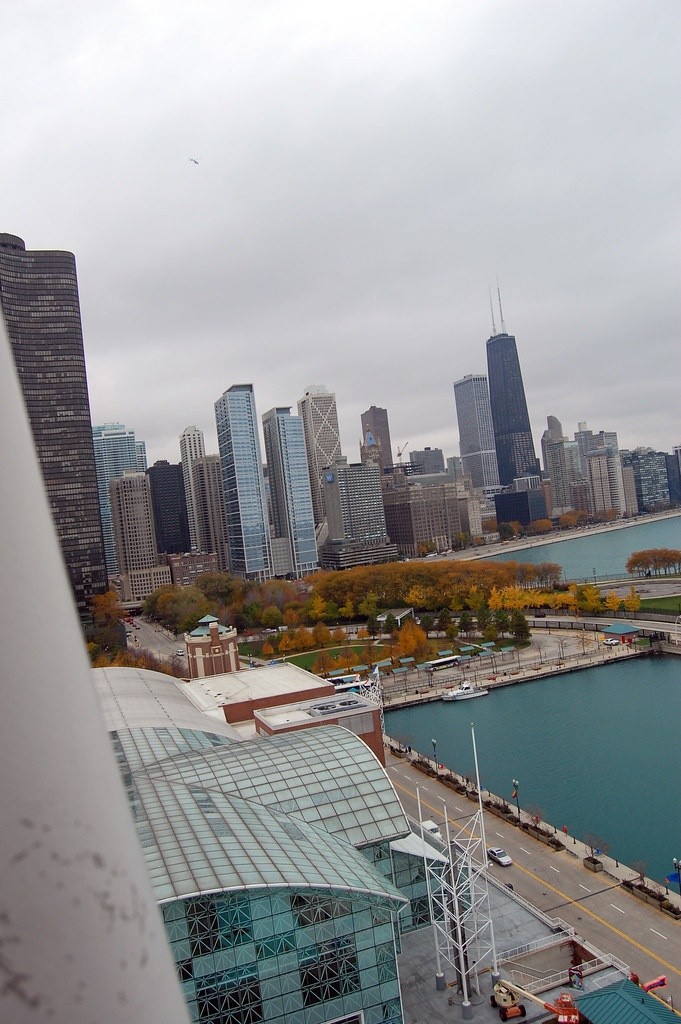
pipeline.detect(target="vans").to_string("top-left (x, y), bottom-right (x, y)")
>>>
top-left (176, 649), bottom-right (185, 656)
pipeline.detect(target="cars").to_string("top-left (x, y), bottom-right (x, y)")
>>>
top-left (487, 846), bottom-right (512, 867)
top-left (603, 639), bottom-right (620, 646)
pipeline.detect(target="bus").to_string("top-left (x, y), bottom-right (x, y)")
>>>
top-left (425, 655), bottom-right (462, 672)
top-left (326, 673), bottom-right (361, 687)
top-left (334, 678), bottom-right (376, 694)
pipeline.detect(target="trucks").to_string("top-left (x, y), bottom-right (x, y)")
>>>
top-left (421, 819), bottom-right (443, 842)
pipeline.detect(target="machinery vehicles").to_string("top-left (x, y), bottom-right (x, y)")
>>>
top-left (489, 978), bottom-right (580, 1024)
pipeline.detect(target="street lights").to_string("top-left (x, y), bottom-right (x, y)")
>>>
top-left (512, 778), bottom-right (520, 813)
top-left (672, 857), bottom-right (681, 897)
top-left (432, 739), bottom-right (437, 762)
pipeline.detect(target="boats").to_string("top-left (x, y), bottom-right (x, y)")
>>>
top-left (440, 680), bottom-right (489, 702)
